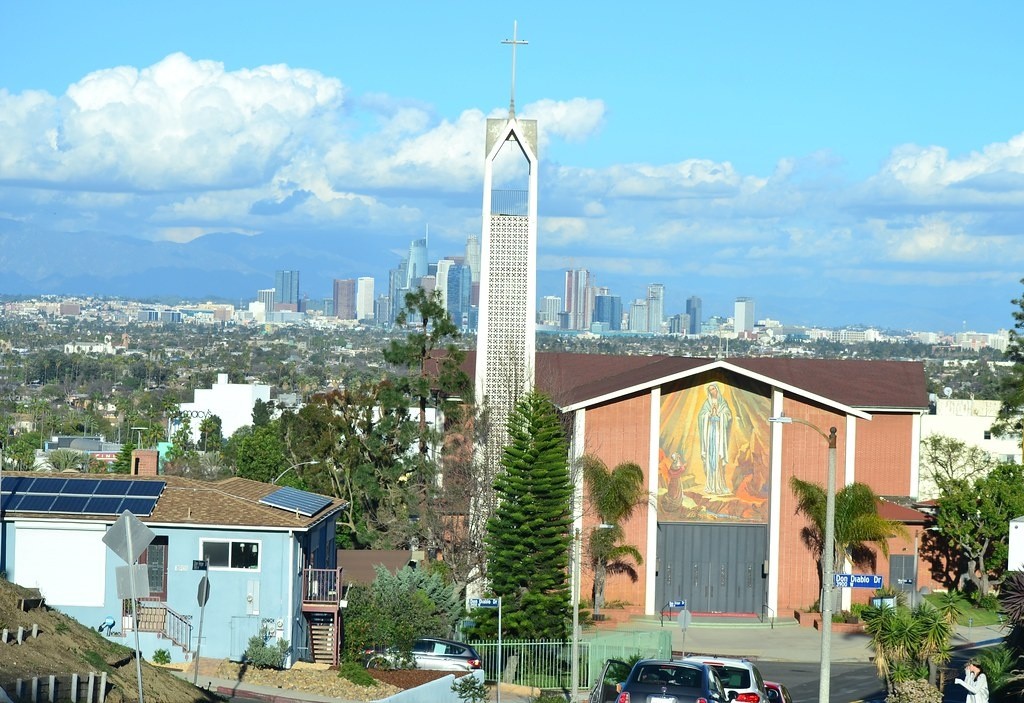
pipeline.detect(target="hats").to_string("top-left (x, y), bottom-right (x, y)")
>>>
top-left (963, 658), bottom-right (981, 666)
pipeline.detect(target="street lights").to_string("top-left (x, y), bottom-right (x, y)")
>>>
top-left (908, 523), bottom-right (944, 608)
top-left (768, 411), bottom-right (840, 703)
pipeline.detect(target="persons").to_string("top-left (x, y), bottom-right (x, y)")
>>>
top-left (616, 669), bottom-right (654, 693)
top-left (954, 657), bottom-right (989, 703)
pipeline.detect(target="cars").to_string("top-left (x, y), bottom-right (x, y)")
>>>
top-left (587, 657), bottom-right (739, 703)
top-left (762, 679), bottom-right (794, 703)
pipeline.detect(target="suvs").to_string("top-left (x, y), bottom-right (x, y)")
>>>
top-left (684, 655), bottom-right (771, 703)
top-left (361, 638), bottom-right (484, 688)
